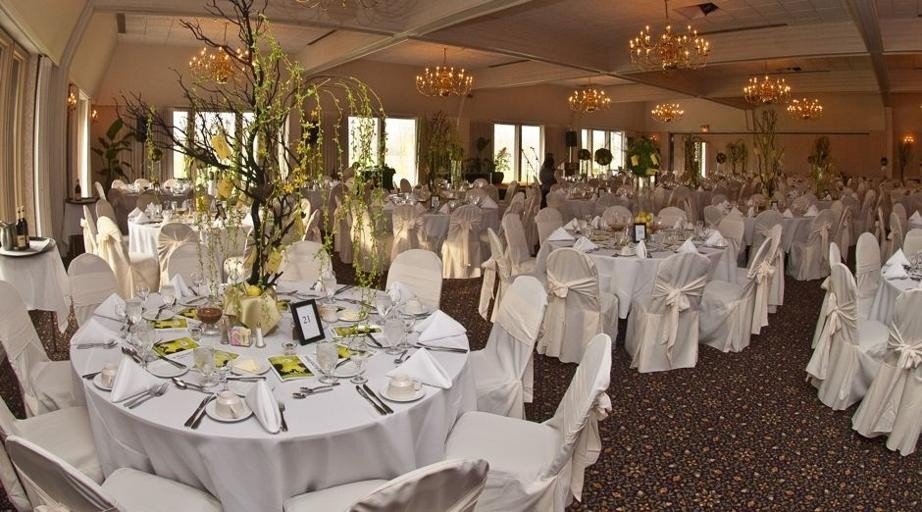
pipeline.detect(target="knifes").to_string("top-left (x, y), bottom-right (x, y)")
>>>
top-left (183, 392), bottom-right (219, 429)
top-left (413, 342), bottom-right (468, 353)
top-left (355, 383), bottom-right (394, 417)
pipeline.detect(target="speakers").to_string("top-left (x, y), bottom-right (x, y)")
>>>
top-left (566, 131), bottom-right (576, 147)
top-left (301, 121), bottom-right (319, 143)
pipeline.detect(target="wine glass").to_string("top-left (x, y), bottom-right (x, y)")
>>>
top-left (115, 268), bottom-right (416, 396)
top-left (579, 214), bottom-right (712, 250)
top-left (145, 197), bottom-right (239, 232)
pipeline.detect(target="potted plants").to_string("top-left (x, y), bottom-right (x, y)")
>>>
top-left (117, 0), bottom-right (394, 337)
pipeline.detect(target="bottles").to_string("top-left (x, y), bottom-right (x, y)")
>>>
top-left (16, 205), bottom-right (30, 250)
top-left (75, 179), bottom-right (81, 201)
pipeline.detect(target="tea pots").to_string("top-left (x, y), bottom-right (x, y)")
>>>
top-left (0, 221), bottom-right (18, 252)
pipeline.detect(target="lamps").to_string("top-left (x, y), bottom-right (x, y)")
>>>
top-left (188, 23), bottom-right (257, 82)
top-left (66, 89), bottom-right (79, 113)
top-left (743, 58), bottom-right (823, 122)
top-left (628, 2), bottom-right (713, 72)
top-left (415, 47), bottom-right (475, 97)
top-left (651, 100), bottom-right (687, 125)
top-left (567, 81), bottom-right (612, 114)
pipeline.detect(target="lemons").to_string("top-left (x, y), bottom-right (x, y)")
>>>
top-left (633, 209), bottom-right (654, 235)
top-left (245, 285), bottom-right (263, 298)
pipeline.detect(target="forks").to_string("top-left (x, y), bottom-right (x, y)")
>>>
top-left (74, 341), bottom-right (118, 350)
top-left (122, 381), bottom-right (169, 410)
top-left (276, 404), bottom-right (290, 431)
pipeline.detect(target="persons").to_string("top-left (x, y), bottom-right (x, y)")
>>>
top-left (539, 156), bottom-right (557, 209)
top-left (340, 161), bottom-right (360, 183)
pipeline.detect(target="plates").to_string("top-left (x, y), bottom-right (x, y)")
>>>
top-left (140, 286), bottom-right (430, 422)
